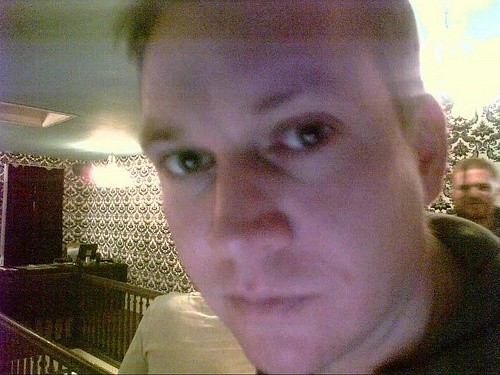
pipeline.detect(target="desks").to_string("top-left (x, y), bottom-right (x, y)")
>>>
top-left (1, 263), bottom-right (128, 318)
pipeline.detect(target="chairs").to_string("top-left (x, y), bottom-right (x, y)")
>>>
top-left (27, 248), bottom-right (55, 265)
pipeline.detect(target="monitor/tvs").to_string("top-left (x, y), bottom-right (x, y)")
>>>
top-left (76, 243), bottom-right (98, 263)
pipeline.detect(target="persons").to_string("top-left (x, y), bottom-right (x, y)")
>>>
top-left (114, 0), bottom-right (500, 375)
top-left (446, 157), bottom-right (500, 237)
top-left (118, 291), bottom-right (258, 375)
